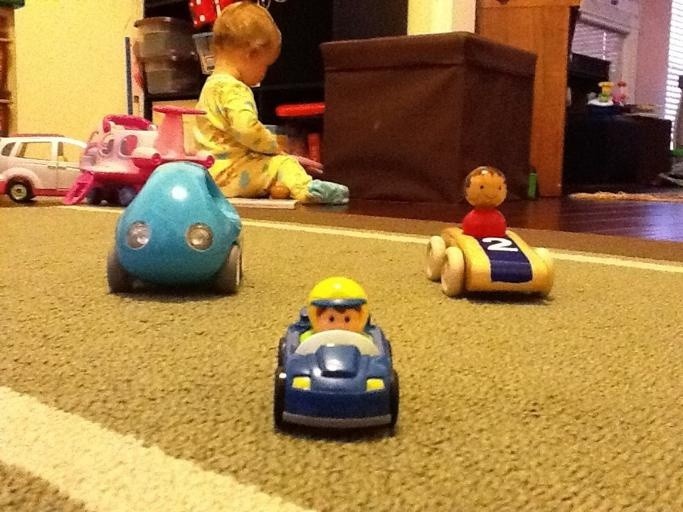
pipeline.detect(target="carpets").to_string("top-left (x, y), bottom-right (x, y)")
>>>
top-left (570, 189), bottom-right (682, 203)
top-left (3, 205), bottom-right (681, 512)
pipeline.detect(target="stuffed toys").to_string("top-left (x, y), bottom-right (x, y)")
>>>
top-left (598, 80), bottom-right (630, 107)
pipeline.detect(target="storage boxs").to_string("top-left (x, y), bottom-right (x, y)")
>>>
top-left (193, 32), bottom-right (221, 70)
top-left (143, 60), bottom-right (192, 93)
top-left (136, 17), bottom-right (189, 58)
top-left (322, 31), bottom-right (538, 205)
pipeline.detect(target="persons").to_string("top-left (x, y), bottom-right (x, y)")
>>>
top-left (191, 2), bottom-right (349, 205)
top-left (424, 166), bottom-right (554, 298)
top-left (275, 276), bottom-right (400, 436)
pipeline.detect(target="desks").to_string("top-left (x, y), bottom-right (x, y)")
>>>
top-left (567, 113), bottom-right (671, 192)
